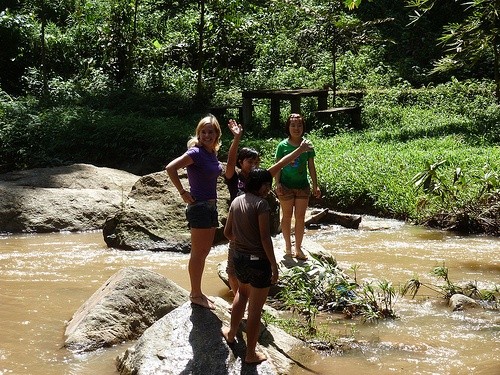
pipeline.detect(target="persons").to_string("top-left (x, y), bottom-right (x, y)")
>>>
top-left (224, 119), bottom-right (315, 313)
top-left (166, 113), bottom-right (222, 310)
top-left (275, 113), bottom-right (321, 260)
top-left (221, 168), bottom-right (279, 364)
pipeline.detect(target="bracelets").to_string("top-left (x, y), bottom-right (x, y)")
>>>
top-left (180, 190), bottom-right (185, 195)
